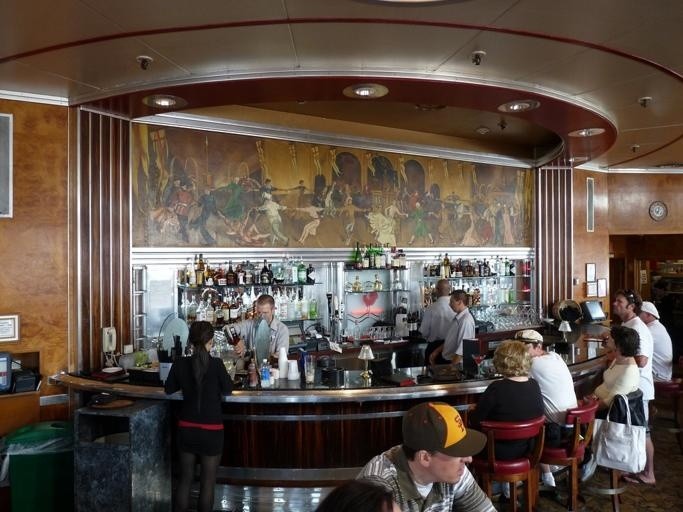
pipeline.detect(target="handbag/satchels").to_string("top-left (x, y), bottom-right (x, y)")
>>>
top-left (591, 419), bottom-right (647, 474)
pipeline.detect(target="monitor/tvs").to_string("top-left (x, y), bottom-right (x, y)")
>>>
top-left (581, 300), bottom-right (606, 319)
top-left (0, 352), bottom-right (11, 393)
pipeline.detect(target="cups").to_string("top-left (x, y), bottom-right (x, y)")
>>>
top-left (304, 355), bottom-right (315, 384)
top-left (223, 359), bottom-right (232, 370)
top-left (277, 346), bottom-right (299, 381)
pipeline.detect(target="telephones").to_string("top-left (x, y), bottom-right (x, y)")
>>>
top-left (102, 326), bottom-right (117, 352)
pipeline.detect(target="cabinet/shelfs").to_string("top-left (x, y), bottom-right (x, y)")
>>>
top-left (175, 276), bottom-right (324, 331)
top-left (342, 263), bottom-right (411, 298)
top-left (417, 271), bottom-right (532, 312)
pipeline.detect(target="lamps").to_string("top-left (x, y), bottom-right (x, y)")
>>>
top-left (557, 320), bottom-right (572, 342)
top-left (356, 344), bottom-right (375, 378)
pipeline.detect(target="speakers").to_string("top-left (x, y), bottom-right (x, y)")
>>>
top-left (0, 114), bottom-right (14, 219)
top-left (586, 176), bottom-right (594, 233)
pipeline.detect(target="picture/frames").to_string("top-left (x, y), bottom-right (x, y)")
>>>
top-left (0, 112), bottom-right (17, 218)
top-left (585, 262), bottom-right (607, 299)
top-left (0, 310), bottom-right (21, 343)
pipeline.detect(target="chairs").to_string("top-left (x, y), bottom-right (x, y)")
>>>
top-left (461, 414), bottom-right (547, 510)
top-left (572, 388), bottom-right (648, 509)
top-left (646, 356), bottom-right (683, 448)
top-left (529, 394), bottom-right (601, 511)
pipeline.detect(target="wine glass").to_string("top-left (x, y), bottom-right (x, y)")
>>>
top-left (471, 354), bottom-right (486, 378)
top-left (471, 303), bottom-right (535, 328)
top-left (368, 325), bottom-right (392, 340)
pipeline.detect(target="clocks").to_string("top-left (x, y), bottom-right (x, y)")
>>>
top-left (648, 199), bottom-right (667, 222)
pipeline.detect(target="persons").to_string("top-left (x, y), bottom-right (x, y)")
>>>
top-left (472, 339), bottom-right (544, 499)
top-left (161, 320), bottom-right (234, 511)
top-left (313, 479), bottom-right (403, 512)
top-left (428, 290), bottom-right (475, 363)
top-left (227, 295), bottom-right (289, 362)
top-left (515, 328), bottom-right (579, 500)
top-left (580, 326), bottom-right (646, 482)
top-left (612, 289), bottom-right (656, 485)
top-left (354, 401), bottom-right (504, 511)
top-left (417, 279), bottom-right (461, 364)
top-left (634, 300), bottom-right (672, 383)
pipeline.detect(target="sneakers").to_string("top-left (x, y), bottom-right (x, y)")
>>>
top-left (581, 454), bottom-right (597, 481)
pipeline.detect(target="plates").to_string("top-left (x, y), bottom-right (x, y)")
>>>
top-left (101, 367), bottom-right (123, 373)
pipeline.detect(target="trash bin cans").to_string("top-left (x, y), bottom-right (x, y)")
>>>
top-left (0, 421), bottom-right (74, 512)
top-left (74, 401), bottom-right (172, 512)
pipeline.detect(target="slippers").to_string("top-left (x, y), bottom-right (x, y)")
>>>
top-left (623, 476), bottom-right (656, 486)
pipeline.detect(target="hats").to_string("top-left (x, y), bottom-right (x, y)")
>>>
top-left (403, 402), bottom-right (487, 456)
top-left (514, 330), bottom-right (544, 343)
top-left (641, 301), bottom-right (660, 319)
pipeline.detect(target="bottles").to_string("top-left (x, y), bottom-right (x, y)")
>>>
top-left (258, 358), bottom-right (270, 388)
top-left (223, 326), bottom-right (246, 356)
top-left (247, 359), bottom-right (257, 389)
top-left (406, 313), bottom-right (418, 337)
top-left (180, 253), bottom-right (317, 325)
top-left (353, 320), bottom-right (360, 340)
top-left (422, 253), bottom-right (516, 308)
top-left (354, 240), bottom-right (405, 269)
top-left (351, 275), bottom-right (362, 292)
top-left (373, 274), bottom-right (382, 290)
